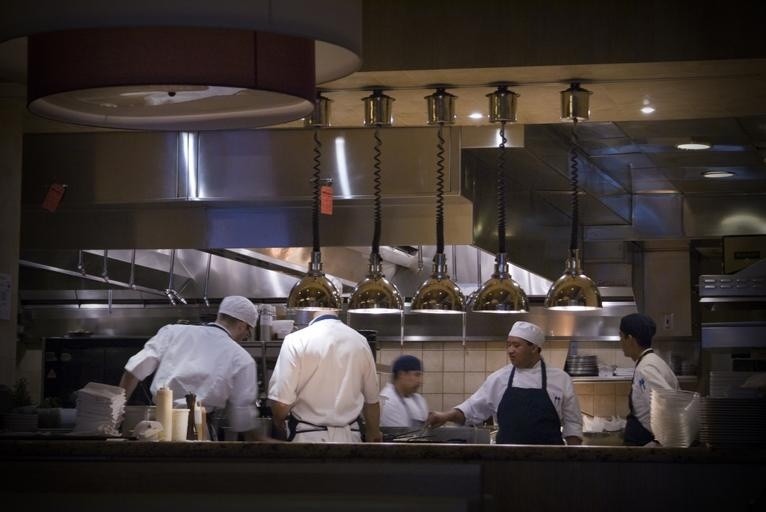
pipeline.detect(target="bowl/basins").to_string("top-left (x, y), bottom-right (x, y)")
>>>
top-left (271, 320), bottom-right (295, 334)
top-left (651, 385), bottom-right (704, 448)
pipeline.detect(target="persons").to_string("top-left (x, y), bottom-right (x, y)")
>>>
top-left (267, 293), bottom-right (384, 442)
top-left (618, 313), bottom-right (682, 446)
top-left (378, 355), bottom-right (429, 427)
top-left (424, 320), bottom-right (585, 445)
top-left (118, 295), bottom-right (268, 440)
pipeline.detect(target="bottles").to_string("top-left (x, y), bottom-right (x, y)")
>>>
top-left (157, 388), bottom-right (210, 441)
top-left (260, 307), bottom-right (275, 341)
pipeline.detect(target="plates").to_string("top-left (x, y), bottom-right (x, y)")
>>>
top-left (5, 413), bottom-right (40, 432)
top-left (697, 396), bottom-right (766, 448)
top-left (565, 355), bottom-right (599, 376)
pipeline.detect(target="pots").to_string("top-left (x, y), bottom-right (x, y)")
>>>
top-left (245, 416), bottom-right (276, 441)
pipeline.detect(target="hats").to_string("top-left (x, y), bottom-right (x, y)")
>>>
top-left (393, 355), bottom-right (421, 371)
top-left (620, 313), bottom-right (656, 337)
top-left (218, 296), bottom-right (258, 328)
top-left (509, 321), bottom-right (544, 347)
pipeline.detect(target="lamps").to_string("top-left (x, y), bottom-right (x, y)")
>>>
top-left (286, 93), bottom-right (343, 311)
top-left (472, 86), bottom-right (530, 314)
top-left (409, 89), bottom-right (467, 314)
top-left (545, 82), bottom-right (603, 311)
top-left (347, 90), bottom-right (405, 315)
